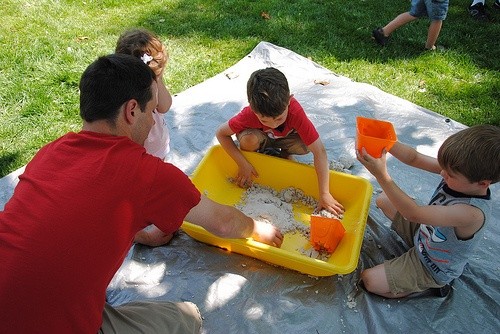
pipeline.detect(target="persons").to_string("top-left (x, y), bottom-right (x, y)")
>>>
top-left (215, 67), bottom-right (346, 220)
top-left (372, 0), bottom-right (500, 52)
top-left (354, 124), bottom-right (500, 300)
top-left (0, 29), bottom-right (284, 334)
